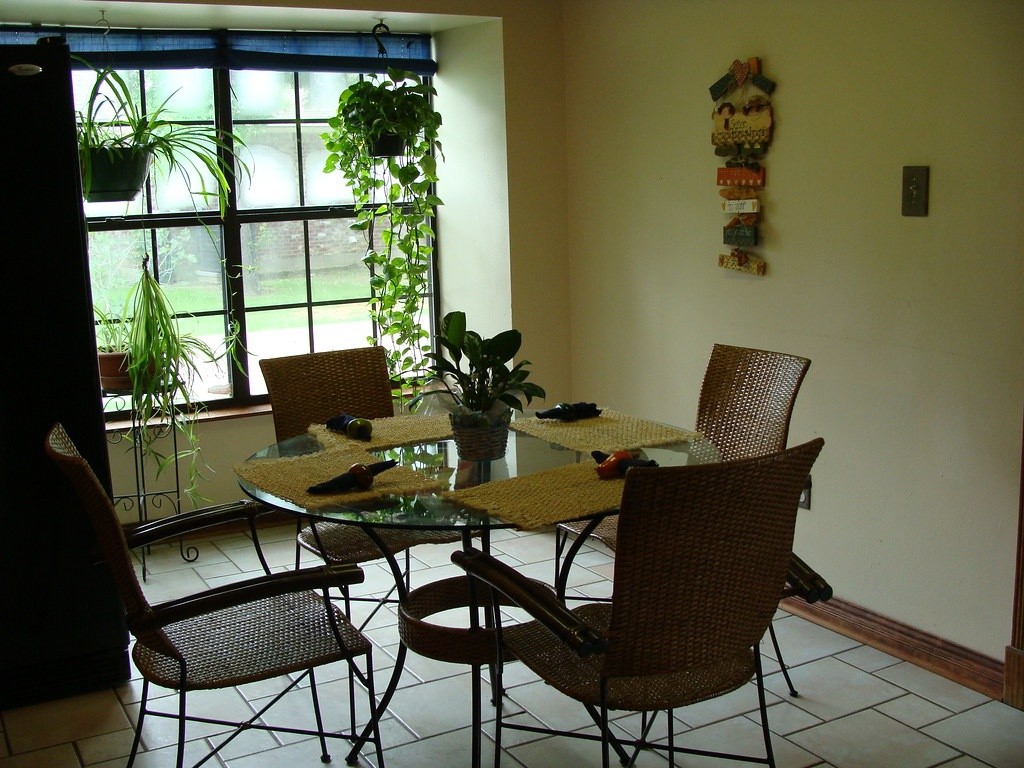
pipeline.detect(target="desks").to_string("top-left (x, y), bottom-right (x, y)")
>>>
top-left (233, 408), bottom-right (721, 767)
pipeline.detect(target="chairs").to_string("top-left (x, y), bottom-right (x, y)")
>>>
top-left (44, 424), bottom-right (387, 768)
top-left (260, 346), bottom-right (496, 689)
top-left (556, 345), bottom-right (818, 696)
top-left (451, 441), bottom-right (825, 768)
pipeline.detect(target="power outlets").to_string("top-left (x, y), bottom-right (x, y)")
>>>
top-left (798, 488), bottom-right (810, 510)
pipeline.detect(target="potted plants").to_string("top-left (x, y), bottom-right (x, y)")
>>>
top-left (66, 53), bottom-right (252, 427)
top-left (423, 303), bottom-right (546, 460)
top-left (326, 64), bottom-right (445, 411)
top-left (86, 280), bottom-right (219, 513)
